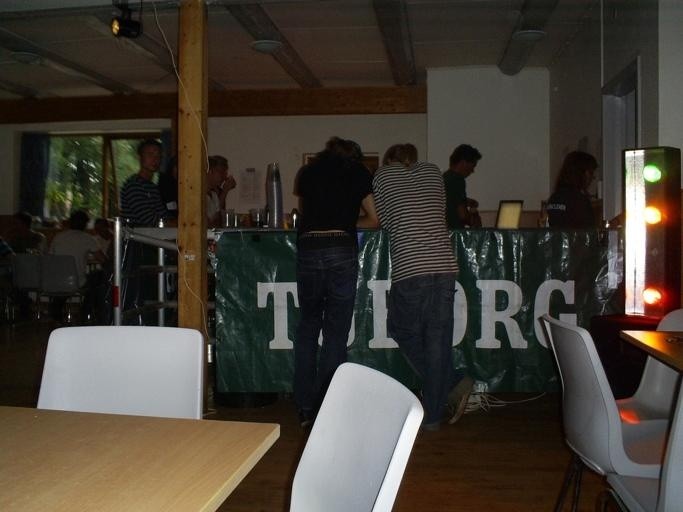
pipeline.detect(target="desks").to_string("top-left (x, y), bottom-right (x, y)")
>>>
top-left (0, 403), bottom-right (280, 511)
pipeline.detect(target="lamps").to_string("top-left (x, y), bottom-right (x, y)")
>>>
top-left (107, 1), bottom-right (144, 39)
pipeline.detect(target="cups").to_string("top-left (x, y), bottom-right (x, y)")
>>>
top-left (31, 216), bottom-right (66, 227)
top-left (248, 208), bottom-right (264, 226)
top-left (221, 209), bottom-right (239, 228)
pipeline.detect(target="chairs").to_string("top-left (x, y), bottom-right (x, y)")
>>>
top-left (34, 325), bottom-right (204, 420)
top-left (0, 252), bottom-right (103, 324)
top-left (539, 308), bottom-right (683, 512)
top-left (287, 361), bottom-right (424, 511)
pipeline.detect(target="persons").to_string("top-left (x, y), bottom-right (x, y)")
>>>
top-left (198, 144), bottom-right (242, 331)
top-left (543, 146), bottom-right (605, 230)
top-left (375, 142), bottom-right (475, 434)
top-left (440, 143), bottom-right (482, 231)
top-left (0, 207), bottom-right (120, 328)
top-left (290, 133), bottom-right (382, 431)
top-left (118, 139), bottom-right (172, 309)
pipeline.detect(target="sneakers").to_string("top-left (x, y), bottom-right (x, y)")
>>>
top-left (298, 407), bottom-right (314, 427)
top-left (422, 422), bottom-right (442, 431)
top-left (446, 377), bottom-right (472, 425)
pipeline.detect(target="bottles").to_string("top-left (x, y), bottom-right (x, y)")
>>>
top-left (157, 218), bottom-right (165, 228)
top-left (265, 161), bottom-right (283, 229)
top-left (290, 208), bottom-right (298, 231)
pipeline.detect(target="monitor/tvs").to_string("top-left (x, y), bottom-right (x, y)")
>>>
top-left (623, 146), bottom-right (681, 319)
top-left (496, 199), bottom-right (523, 232)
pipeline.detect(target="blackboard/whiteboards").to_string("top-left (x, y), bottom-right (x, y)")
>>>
top-left (427, 64), bottom-right (551, 213)
